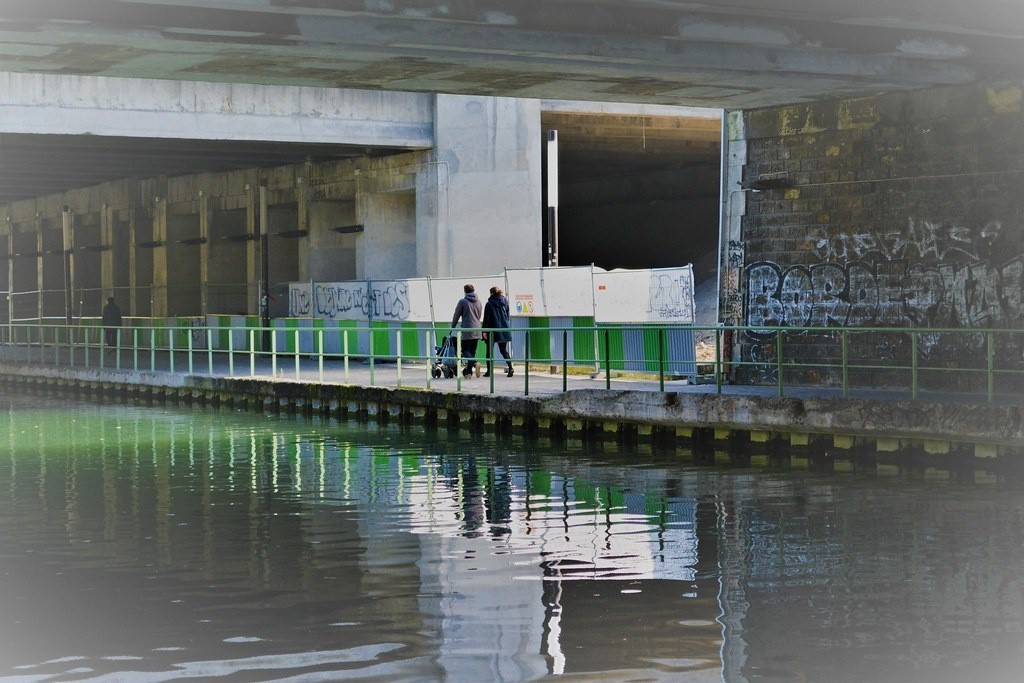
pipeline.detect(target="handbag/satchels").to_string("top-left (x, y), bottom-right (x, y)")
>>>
top-left (442, 345), bottom-right (459, 367)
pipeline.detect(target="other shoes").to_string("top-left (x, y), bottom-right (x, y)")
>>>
top-left (463, 373), bottom-right (472, 381)
top-left (507, 369), bottom-right (515, 378)
top-left (475, 362), bottom-right (482, 378)
top-left (111, 351), bottom-right (117, 356)
top-left (483, 371), bottom-right (493, 377)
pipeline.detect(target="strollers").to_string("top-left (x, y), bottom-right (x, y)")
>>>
top-left (431, 331), bottom-right (457, 379)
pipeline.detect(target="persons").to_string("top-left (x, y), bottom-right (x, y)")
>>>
top-left (103, 297), bottom-right (121, 354)
top-left (481, 287), bottom-right (515, 377)
top-left (451, 285), bottom-right (482, 379)
